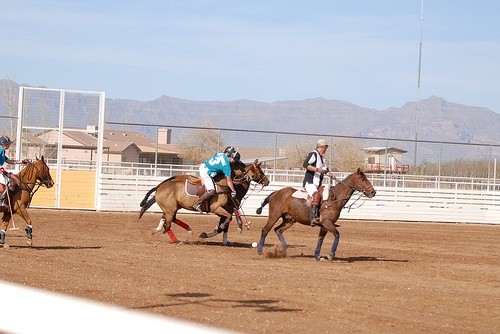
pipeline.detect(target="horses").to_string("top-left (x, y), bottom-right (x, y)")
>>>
top-left (138, 158), bottom-right (271, 247)
top-left (255, 168), bottom-right (378, 262)
top-left (1, 156), bottom-right (56, 245)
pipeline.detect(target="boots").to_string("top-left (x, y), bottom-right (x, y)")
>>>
top-left (309, 205), bottom-right (320, 227)
top-left (192, 189), bottom-right (215, 215)
top-left (0, 192), bottom-right (12, 207)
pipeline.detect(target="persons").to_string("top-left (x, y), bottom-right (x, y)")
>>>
top-left (303, 139), bottom-right (336, 227)
top-left (0, 136), bottom-right (30, 206)
top-left (192, 146), bottom-right (241, 213)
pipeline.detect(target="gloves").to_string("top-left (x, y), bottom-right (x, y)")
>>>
top-left (231, 191), bottom-right (236, 198)
top-left (318, 167), bottom-right (327, 174)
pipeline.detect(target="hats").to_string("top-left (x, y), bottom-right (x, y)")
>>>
top-left (317, 139), bottom-right (328, 147)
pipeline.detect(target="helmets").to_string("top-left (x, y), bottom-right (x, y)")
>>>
top-left (0, 136), bottom-right (13, 145)
top-left (225, 146), bottom-right (234, 153)
top-left (231, 151), bottom-right (241, 163)
